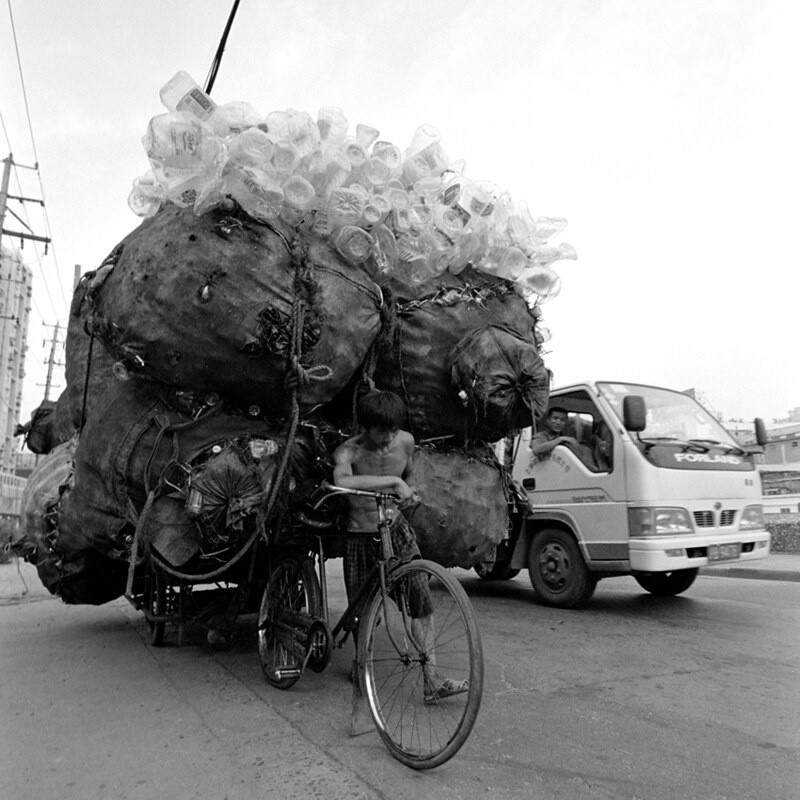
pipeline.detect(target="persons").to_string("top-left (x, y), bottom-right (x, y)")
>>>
top-left (532, 406), bottom-right (581, 456)
top-left (602, 426), bottom-right (611, 469)
top-left (333, 391), bottom-right (470, 702)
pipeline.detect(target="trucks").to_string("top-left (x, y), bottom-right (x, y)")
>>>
top-left (473, 380), bottom-right (772, 609)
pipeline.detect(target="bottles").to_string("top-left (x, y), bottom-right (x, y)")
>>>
top-left (56, 70), bottom-right (580, 542)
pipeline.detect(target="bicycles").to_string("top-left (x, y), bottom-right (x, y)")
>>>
top-left (255, 481), bottom-right (484, 771)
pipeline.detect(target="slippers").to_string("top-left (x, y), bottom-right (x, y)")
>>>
top-left (425, 677), bottom-right (469, 703)
top-left (349, 661), bottom-right (361, 684)
